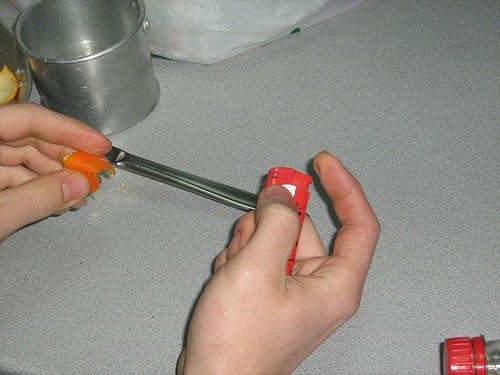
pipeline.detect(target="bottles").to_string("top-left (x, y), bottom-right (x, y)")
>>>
top-left (443, 335), bottom-right (500, 375)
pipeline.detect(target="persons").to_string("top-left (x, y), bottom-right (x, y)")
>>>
top-left (0, 102), bottom-right (381, 374)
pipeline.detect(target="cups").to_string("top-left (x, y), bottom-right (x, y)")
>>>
top-left (15, 0), bottom-right (161, 135)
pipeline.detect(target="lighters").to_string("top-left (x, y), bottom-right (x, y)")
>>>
top-left (262, 166), bottom-right (312, 278)
